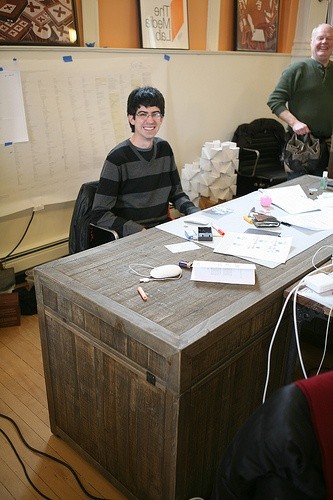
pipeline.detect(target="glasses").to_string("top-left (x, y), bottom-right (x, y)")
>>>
top-left (136, 111), bottom-right (162, 121)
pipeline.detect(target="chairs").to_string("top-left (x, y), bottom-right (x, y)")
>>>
top-left (234, 118), bottom-right (288, 197)
top-left (68, 180), bottom-right (120, 253)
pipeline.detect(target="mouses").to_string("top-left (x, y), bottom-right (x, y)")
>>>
top-left (149, 264), bottom-right (182, 279)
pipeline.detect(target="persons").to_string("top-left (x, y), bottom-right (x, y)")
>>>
top-left (267, 23), bottom-right (333, 181)
top-left (91, 87), bottom-right (201, 237)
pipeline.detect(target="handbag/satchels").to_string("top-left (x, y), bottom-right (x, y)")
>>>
top-left (282, 132), bottom-right (329, 178)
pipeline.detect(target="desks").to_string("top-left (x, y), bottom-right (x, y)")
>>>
top-left (33, 174), bottom-right (333, 498)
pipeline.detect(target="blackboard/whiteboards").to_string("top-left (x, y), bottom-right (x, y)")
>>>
top-left (0, 45), bottom-right (333, 261)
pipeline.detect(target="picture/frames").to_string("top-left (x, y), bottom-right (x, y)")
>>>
top-left (0, 0), bottom-right (84, 47)
top-left (138, 0), bottom-right (190, 50)
top-left (234, 0), bottom-right (280, 53)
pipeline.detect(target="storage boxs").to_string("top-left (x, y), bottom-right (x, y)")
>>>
top-left (0, 292), bottom-right (20, 328)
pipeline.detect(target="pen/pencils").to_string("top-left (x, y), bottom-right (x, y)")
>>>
top-left (211, 221), bottom-right (226, 236)
